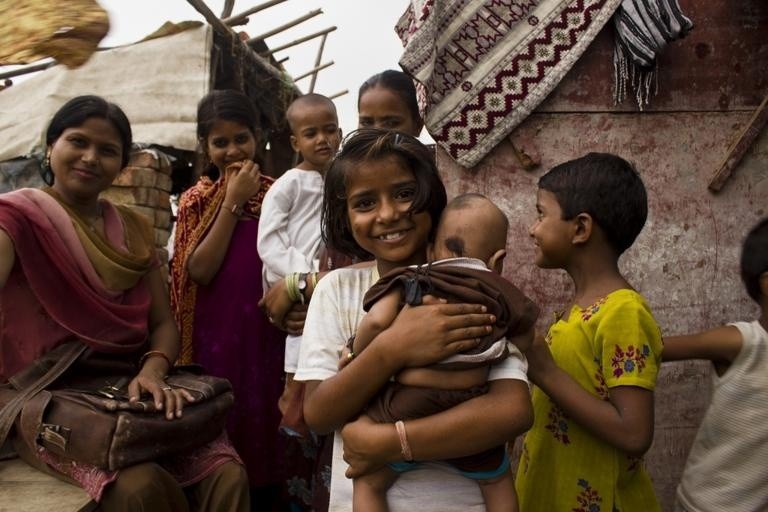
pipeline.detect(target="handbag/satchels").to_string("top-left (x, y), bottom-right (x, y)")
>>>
top-left (0, 341), bottom-right (235, 472)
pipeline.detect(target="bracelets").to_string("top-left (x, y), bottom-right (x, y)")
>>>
top-left (136, 350), bottom-right (174, 373)
top-left (394, 420), bottom-right (413, 462)
top-left (284, 271), bottom-right (318, 303)
top-left (346, 334), bottom-right (355, 359)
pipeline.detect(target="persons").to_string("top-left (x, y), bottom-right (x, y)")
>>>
top-left (257, 70), bottom-right (425, 512)
top-left (338, 192), bottom-right (542, 512)
top-left (1, 94), bottom-right (254, 511)
top-left (169, 88), bottom-right (286, 512)
top-left (511, 151), bottom-right (665, 511)
top-left (662, 217), bottom-right (768, 512)
top-left (256, 93), bottom-right (344, 410)
top-left (292, 124), bottom-right (542, 512)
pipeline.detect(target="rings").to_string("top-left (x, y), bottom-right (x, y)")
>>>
top-left (161, 385), bottom-right (173, 391)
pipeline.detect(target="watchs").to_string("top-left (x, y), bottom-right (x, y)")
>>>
top-left (221, 201), bottom-right (244, 218)
